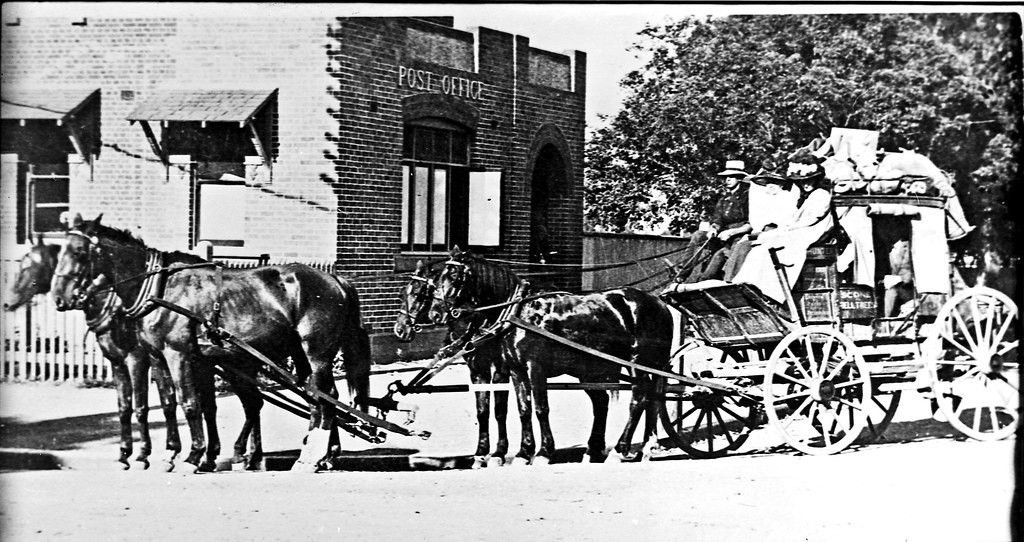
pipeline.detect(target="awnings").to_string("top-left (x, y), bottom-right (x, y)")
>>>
top-left (127, 89), bottom-right (277, 163)
top-left (1, 87), bottom-right (100, 163)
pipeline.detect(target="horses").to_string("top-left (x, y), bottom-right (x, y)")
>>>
top-left (392, 245), bottom-right (673, 469)
top-left (0, 212), bottom-right (372, 472)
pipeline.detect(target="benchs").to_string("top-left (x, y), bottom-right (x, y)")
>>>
top-left (806, 227), bottom-right (848, 265)
top-left (880, 292), bottom-right (949, 338)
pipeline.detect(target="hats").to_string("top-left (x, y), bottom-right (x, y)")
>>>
top-left (786, 155), bottom-right (825, 180)
top-left (717, 160), bottom-right (749, 177)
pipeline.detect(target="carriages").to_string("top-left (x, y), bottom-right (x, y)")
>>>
top-left (0, 192), bottom-right (1018, 474)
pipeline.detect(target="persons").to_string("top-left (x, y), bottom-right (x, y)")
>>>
top-left (677, 160), bottom-right (749, 282)
top-left (878, 217), bottom-right (912, 318)
top-left (734, 156), bottom-right (833, 303)
top-left (530, 225), bottom-right (547, 255)
